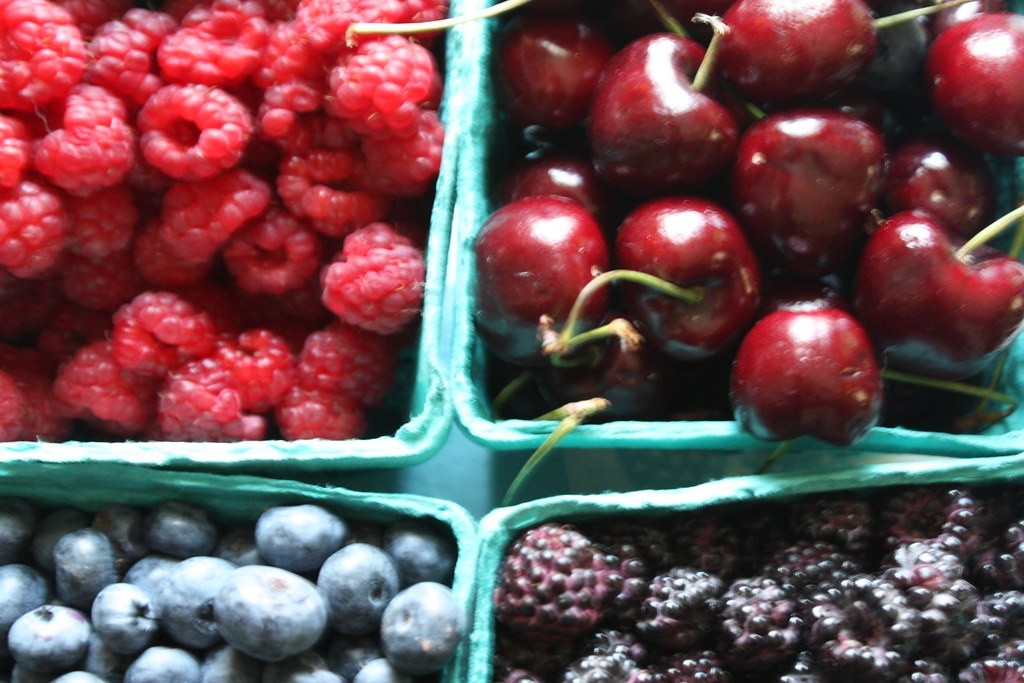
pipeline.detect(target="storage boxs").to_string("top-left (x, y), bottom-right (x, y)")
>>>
top-left (0, 0), bottom-right (1024, 683)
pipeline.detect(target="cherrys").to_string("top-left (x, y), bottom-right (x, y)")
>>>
top-left (347, 0), bottom-right (1024, 498)
